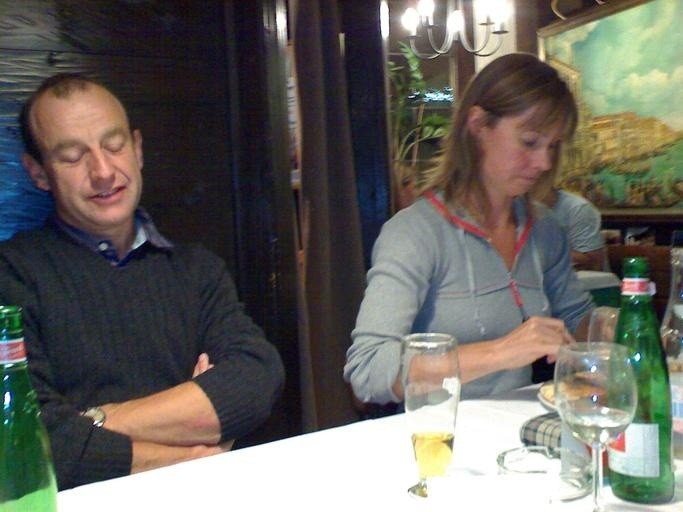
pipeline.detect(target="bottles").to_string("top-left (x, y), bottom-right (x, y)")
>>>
top-left (656, 244), bottom-right (683, 462)
top-left (0, 303), bottom-right (59, 510)
top-left (602, 253), bottom-right (675, 507)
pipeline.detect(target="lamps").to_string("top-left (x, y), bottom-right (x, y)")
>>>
top-left (403, 1), bottom-right (511, 62)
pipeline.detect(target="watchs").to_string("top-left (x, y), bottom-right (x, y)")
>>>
top-left (82, 405), bottom-right (107, 429)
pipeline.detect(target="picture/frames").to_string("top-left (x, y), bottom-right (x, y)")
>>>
top-left (533, 0), bottom-right (683, 227)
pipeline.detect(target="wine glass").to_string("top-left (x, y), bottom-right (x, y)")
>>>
top-left (551, 340), bottom-right (640, 511)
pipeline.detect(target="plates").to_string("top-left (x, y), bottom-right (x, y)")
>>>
top-left (537, 389), bottom-right (555, 412)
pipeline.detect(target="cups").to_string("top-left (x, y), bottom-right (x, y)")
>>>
top-left (399, 330), bottom-right (465, 499)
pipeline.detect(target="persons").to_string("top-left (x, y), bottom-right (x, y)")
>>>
top-left (1, 72), bottom-right (289, 492)
top-left (543, 186), bottom-right (612, 271)
top-left (340, 52), bottom-right (672, 413)
